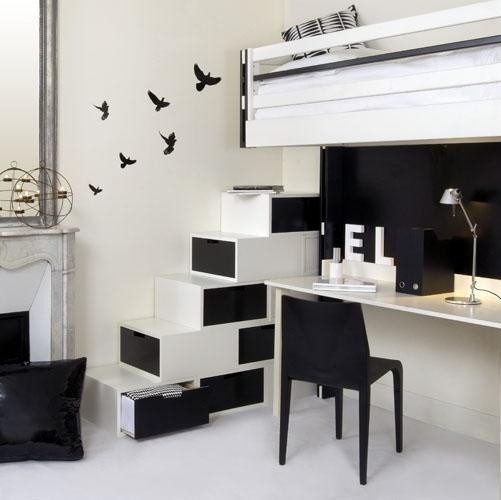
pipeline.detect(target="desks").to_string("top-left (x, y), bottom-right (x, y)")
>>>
top-left (2, 224), bottom-right (79, 363)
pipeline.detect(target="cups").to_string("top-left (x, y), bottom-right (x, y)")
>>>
top-left (329, 263), bottom-right (344, 286)
top-left (348, 257), bottom-right (362, 277)
top-left (322, 259), bottom-right (336, 280)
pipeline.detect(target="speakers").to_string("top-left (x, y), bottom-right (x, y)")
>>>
top-left (396, 227), bottom-right (454, 295)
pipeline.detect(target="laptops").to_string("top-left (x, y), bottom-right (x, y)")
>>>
top-left (313, 276), bottom-right (377, 293)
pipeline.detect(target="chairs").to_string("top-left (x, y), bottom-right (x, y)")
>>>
top-left (279, 295), bottom-right (402, 486)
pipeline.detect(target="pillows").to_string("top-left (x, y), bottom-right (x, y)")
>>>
top-left (281, 4), bottom-right (365, 61)
top-left (0, 356), bottom-right (86, 463)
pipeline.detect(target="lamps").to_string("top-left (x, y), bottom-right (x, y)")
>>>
top-left (439, 188), bottom-right (480, 305)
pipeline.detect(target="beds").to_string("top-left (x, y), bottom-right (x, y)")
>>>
top-left (239, 1), bottom-right (500, 148)
